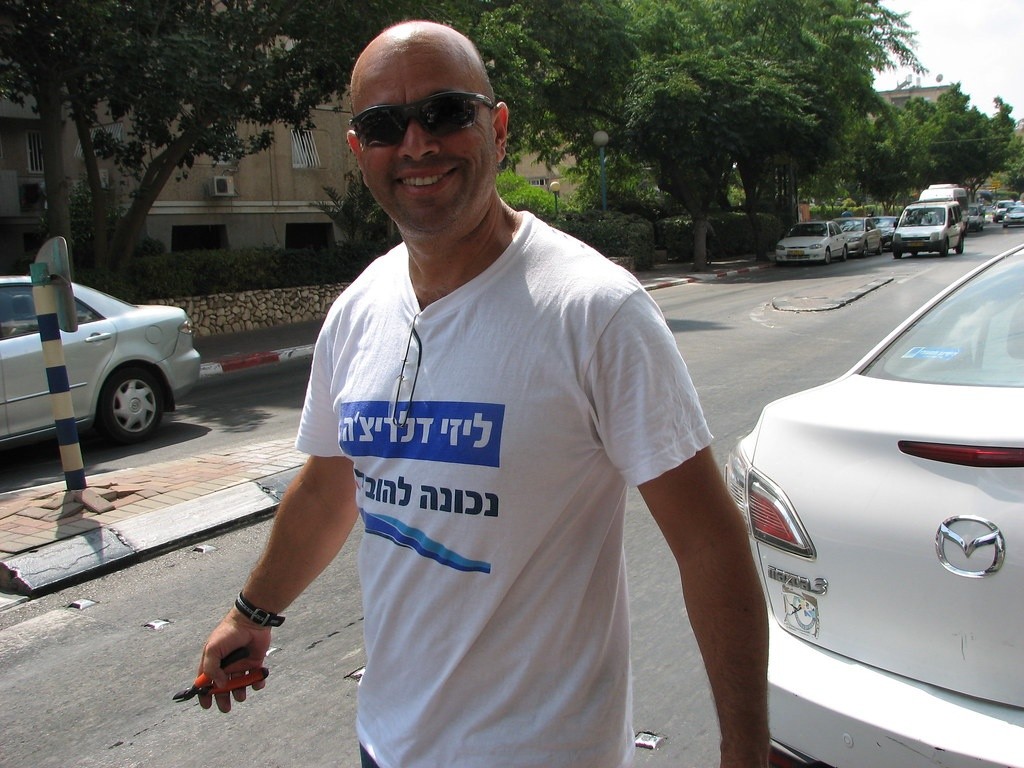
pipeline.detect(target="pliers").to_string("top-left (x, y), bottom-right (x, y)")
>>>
top-left (172, 646), bottom-right (270, 703)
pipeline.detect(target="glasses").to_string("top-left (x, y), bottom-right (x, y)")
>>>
top-left (347, 91), bottom-right (494, 149)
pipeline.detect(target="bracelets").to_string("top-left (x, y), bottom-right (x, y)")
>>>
top-left (235, 590), bottom-right (285, 628)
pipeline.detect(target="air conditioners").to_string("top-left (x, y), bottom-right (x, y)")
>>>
top-left (209, 175), bottom-right (235, 198)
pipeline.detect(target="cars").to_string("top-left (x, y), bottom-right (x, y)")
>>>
top-left (968, 199), bottom-right (1024, 233)
top-left (831, 216), bottom-right (883, 259)
top-left (0, 274), bottom-right (202, 452)
top-left (718, 244), bottom-right (1023, 768)
top-left (872, 214), bottom-right (901, 252)
top-left (774, 220), bottom-right (848, 267)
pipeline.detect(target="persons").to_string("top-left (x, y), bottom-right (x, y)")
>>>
top-left (841, 208), bottom-right (853, 217)
top-left (193, 19), bottom-right (768, 768)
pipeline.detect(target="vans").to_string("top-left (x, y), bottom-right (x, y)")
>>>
top-left (891, 199), bottom-right (965, 259)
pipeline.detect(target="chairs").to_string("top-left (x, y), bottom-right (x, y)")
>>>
top-left (12, 294), bottom-right (37, 320)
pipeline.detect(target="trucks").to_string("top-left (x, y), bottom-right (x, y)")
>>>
top-left (919, 183), bottom-right (969, 237)
